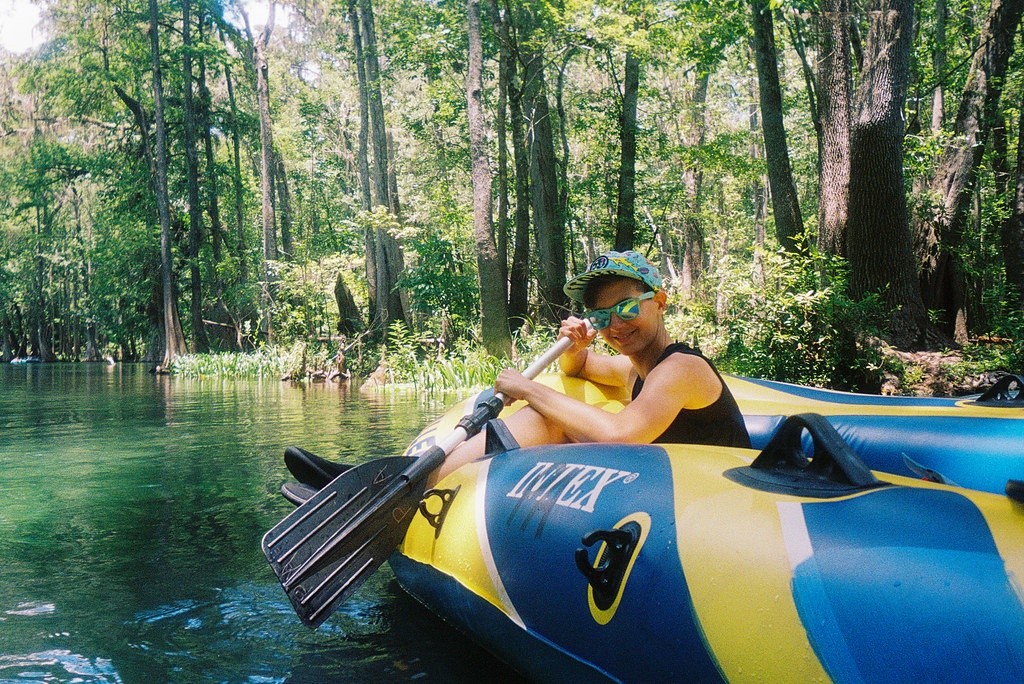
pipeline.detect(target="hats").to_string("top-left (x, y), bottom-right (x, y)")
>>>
top-left (563, 249), bottom-right (666, 304)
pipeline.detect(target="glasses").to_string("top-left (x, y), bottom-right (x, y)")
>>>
top-left (586, 290), bottom-right (657, 330)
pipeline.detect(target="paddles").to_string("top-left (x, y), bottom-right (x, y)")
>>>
top-left (258, 313), bottom-right (609, 631)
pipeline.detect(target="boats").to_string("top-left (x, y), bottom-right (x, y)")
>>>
top-left (375, 366), bottom-right (1024, 684)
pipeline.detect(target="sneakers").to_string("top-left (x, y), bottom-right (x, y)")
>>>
top-left (284, 447), bottom-right (360, 489)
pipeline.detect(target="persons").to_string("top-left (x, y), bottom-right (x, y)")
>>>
top-left (283, 249), bottom-right (754, 491)
